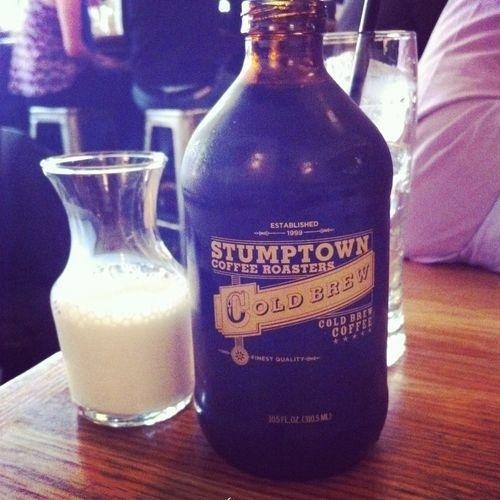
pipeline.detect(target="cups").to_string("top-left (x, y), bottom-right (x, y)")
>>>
top-left (321, 29), bottom-right (419, 366)
top-left (41, 152), bottom-right (193, 428)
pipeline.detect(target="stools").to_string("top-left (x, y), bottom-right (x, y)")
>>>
top-left (142, 109), bottom-right (208, 230)
top-left (28, 106), bottom-right (79, 157)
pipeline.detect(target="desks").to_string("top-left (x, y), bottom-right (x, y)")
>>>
top-left (0, 257), bottom-right (500, 495)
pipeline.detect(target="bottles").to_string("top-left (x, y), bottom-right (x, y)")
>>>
top-left (184, 1), bottom-right (393, 481)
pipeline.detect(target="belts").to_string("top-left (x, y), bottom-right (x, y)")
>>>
top-left (135, 79), bottom-right (210, 100)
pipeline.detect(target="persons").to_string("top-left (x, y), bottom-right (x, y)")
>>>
top-left (119, 1), bottom-right (237, 191)
top-left (13, 0), bottom-right (131, 164)
top-left (406, 0), bottom-right (500, 272)
